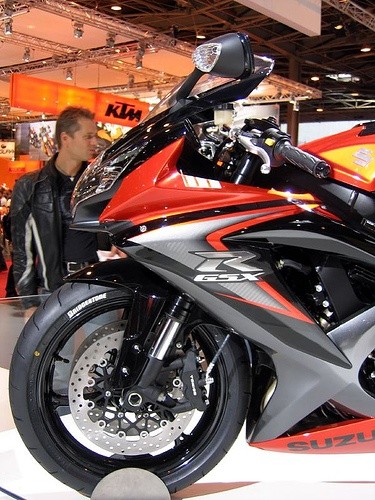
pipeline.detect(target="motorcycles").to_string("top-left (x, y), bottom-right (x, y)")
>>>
top-left (8, 33), bottom-right (375, 498)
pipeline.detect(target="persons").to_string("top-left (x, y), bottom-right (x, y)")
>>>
top-left (10, 107), bottom-right (99, 324)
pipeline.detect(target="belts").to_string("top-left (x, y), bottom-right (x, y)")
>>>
top-left (64, 257), bottom-right (99, 274)
